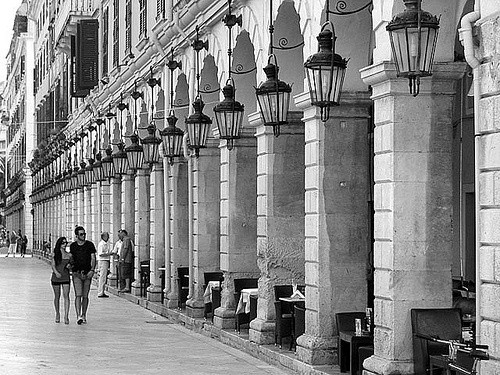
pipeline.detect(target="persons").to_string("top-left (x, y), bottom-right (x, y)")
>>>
top-left (97, 229), bottom-right (134, 298)
top-left (50, 237), bottom-right (75, 324)
top-left (0, 226), bottom-right (28, 258)
top-left (69, 227), bottom-right (96, 325)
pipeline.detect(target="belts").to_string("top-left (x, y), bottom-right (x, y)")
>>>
top-left (77, 270), bottom-right (85, 274)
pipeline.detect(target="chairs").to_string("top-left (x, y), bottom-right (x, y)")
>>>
top-left (273, 284), bottom-right (296, 351)
top-left (335, 312), bottom-right (366, 365)
top-left (139, 261), bottom-right (258, 332)
top-left (411, 308), bottom-right (463, 375)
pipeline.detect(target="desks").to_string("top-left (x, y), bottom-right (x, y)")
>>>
top-left (279, 298), bottom-right (306, 351)
top-left (339, 331), bottom-right (374, 375)
top-left (203, 281), bottom-right (220, 324)
top-left (236, 288), bottom-right (258, 334)
top-left (427, 336), bottom-right (477, 375)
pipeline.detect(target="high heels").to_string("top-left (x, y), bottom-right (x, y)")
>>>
top-left (64, 316), bottom-right (70, 324)
top-left (55, 313), bottom-right (60, 323)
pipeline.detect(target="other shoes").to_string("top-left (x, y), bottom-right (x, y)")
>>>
top-left (98, 294), bottom-right (109, 297)
top-left (121, 288), bottom-right (130, 293)
top-left (80, 315), bottom-right (87, 323)
top-left (77, 318), bottom-right (83, 325)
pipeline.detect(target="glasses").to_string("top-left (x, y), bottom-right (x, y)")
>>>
top-left (80, 233), bottom-right (86, 236)
top-left (61, 242), bottom-right (68, 244)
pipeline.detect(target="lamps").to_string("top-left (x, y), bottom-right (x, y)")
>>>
top-left (304, 0), bottom-right (373, 122)
top-left (0, 47), bottom-right (189, 216)
top-left (185, 25), bottom-right (221, 158)
top-left (385, 0), bottom-right (442, 97)
top-left (213, 0), bottom-right (256, 150)
top-left (253, 0), bottom-right (304, 137)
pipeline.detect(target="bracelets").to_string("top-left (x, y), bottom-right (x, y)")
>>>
top-left (121, 258), bottom-right (124, 260)
top-left (90, 270), bottom-right (94, 272)
